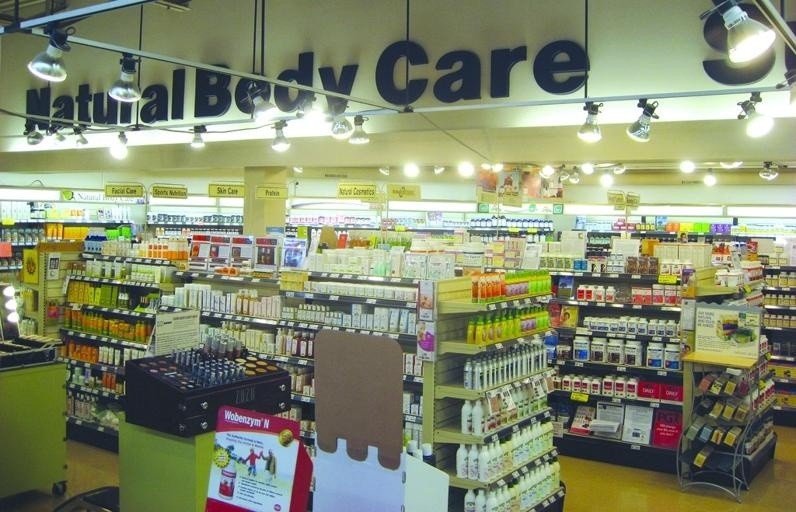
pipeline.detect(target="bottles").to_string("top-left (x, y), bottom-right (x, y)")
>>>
top-left (453, 399), bottom-right (486, 438)
top-left (562, 374), bottom-right (639, 400)
top-left (455, 418), bottom-right (553, 482)
top-left (462, 345), bottom-right (548, 391)
top-left (67, 390), bottom-right (99, 423)
top-left (465, 306), bottom-right (552, 345)
top-left (234, 285), bottom-right (258, 315)
top-left (469, 270), bottom-right (553, 302)
top-left (275, 327), bottom-right (315, 358)
top-left (220, 320), bottom-right (247, 349)
top-left (760, 272), bottom-right (795, 329)
top-left (279, 301), bottom-right (342, 326)
top-left (576, 284), bottom-right (616, 302)
top-left (572, 335), bottom-right (682, 372)
top-left (463, 459), bottom-right (560, 512)
top-left (617, 314), bottom-right (681, 338)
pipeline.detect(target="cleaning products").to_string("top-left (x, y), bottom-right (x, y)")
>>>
top-left (455, 269), bottom-right (562, 512)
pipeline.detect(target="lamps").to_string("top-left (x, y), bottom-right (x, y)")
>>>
top-left (624, 103), bottom-right (656, 144)
top-left (348, 112), bottom-right (369, 145)
top-left (713, 2), bottom-right (777, 63)
top-left (28, 123), bottom-right (45, 145)
top-left (247, 87), bottom-right (277, 120)
top-left (331, 100), bottom-right (354, 140)
top-left (27, 30), bottom-right (72, 83)
top-left (758, 162), bottom-right (779, 181)
top-left (558, 164), bottom-right (570, 181)
top-left (783, 69), bottom-right (796, 105)
top-left (273, 123), bottom-right (291, 153)
top-left (577, 104), bottom-right (602, 145)
top-left (110, 54), bottom-right (141, 103)
top-left (191, 133), bottom-right (204, 148)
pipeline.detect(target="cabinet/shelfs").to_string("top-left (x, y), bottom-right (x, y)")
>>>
top-left (0, 215), bottom-right (796, 512)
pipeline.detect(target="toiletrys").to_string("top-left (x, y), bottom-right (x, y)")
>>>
top-left (54, 263), bottom-right (424, 454)
